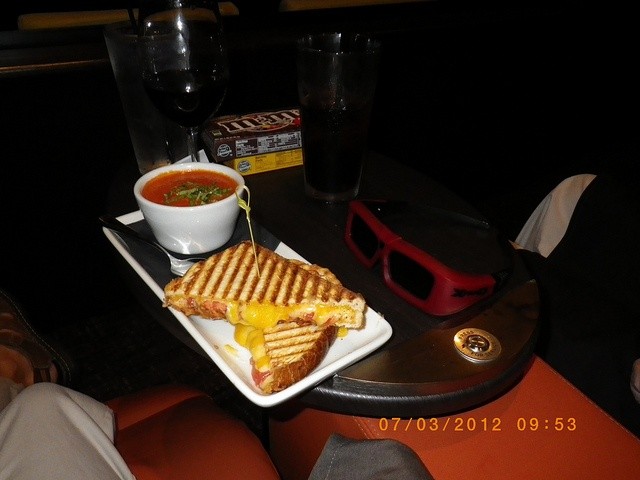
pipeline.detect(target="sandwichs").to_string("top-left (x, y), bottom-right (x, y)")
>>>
top-left (245, 257), bottom-right (343, 395)
top-left (160, 238), bottom-right (366, 331)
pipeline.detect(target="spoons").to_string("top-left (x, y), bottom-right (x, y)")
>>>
top-left (95, 212), bottom-right (206, 277)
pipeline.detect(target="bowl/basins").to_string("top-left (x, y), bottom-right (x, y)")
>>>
top-left (133, 160), bottom-right (246, 256)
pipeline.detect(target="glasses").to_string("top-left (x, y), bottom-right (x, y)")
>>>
top-left (344, 199), bottom-right (515, 317)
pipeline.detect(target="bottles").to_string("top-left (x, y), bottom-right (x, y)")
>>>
top-left (293, 30), bottom-right (383, 201)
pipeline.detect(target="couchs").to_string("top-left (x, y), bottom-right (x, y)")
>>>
top-left (1, 56), bottom-right (234, 395)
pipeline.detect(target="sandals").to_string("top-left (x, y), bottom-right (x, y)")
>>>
top-left (1, 289), bottom-right (77, 387)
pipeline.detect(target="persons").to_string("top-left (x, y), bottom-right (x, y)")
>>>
top-left (0, 382), bottom-right (136, 480)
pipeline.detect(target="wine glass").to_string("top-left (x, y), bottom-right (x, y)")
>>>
top-left (140, 9), bottom-right (229, 162)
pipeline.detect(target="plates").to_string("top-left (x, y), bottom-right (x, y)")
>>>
top-left (102, 209), bottom-right (393, 409)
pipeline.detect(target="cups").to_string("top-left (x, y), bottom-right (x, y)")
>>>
top-left (102, 20), bottom-right (191, 176)
